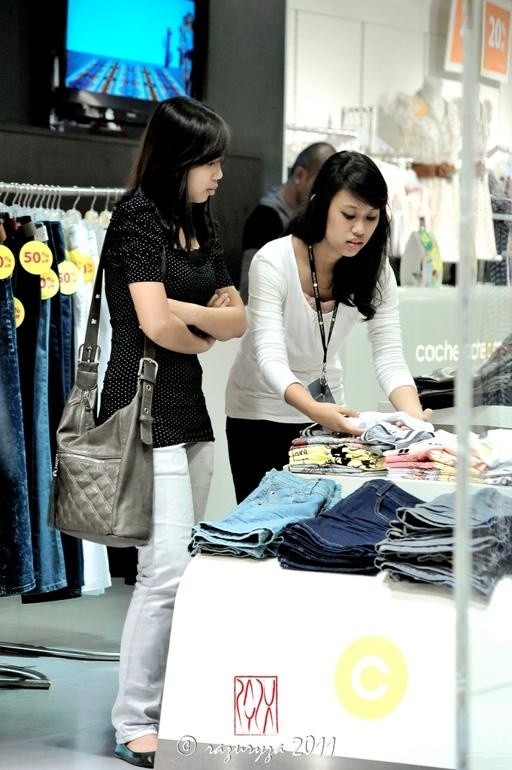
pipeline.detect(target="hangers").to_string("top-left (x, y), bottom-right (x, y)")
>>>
top-left (0, 182), bottom-right (126, 251)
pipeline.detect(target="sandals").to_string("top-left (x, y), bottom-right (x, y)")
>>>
top-left (113, 743), bottom-right (156, 769)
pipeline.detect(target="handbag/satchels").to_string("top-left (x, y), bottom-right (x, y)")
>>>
top-left (46, 382), bottom-right (154, 548)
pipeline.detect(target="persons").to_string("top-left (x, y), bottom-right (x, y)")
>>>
top-left (376, 76), bottom-right (503, 263)
top-left (239, 143), bottom-right (337, 305)
top-left (224, 151), bottom-right (433, 505)
top-left (96, 96), bottom-right (248, 768)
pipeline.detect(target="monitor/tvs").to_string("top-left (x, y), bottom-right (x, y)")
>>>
top-left (52, 0), bottom-right (206, 127)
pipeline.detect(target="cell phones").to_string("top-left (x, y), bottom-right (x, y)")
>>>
top-left (307, 376), bottom-right (336, 403)
